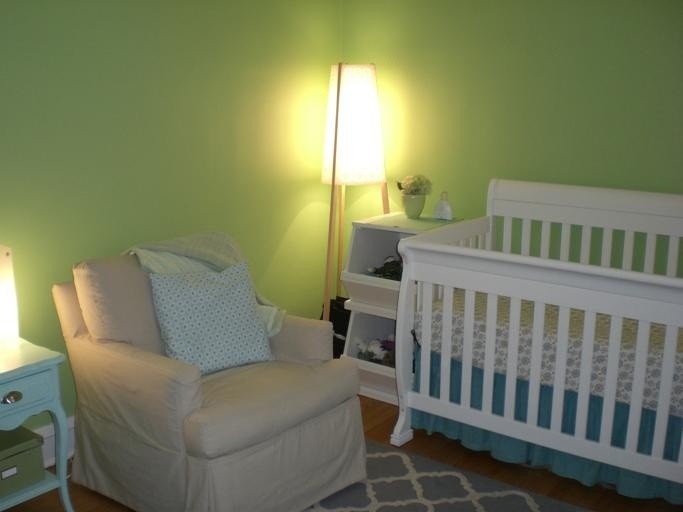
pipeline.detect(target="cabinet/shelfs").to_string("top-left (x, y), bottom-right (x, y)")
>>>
top-left (339, 210), bottom-right (465, 408)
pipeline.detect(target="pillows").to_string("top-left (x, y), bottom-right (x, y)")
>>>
top-left (148, 258), bottom-right (273, 378)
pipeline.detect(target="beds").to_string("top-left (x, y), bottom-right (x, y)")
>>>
top-left (389, 177), bottom-right (682, 486)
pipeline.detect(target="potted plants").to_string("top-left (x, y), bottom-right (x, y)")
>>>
top-left (394, 174), bottom-right (433, 219)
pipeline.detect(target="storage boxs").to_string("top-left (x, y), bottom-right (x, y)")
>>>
top-left (0, 424), bottom-right (45, 499)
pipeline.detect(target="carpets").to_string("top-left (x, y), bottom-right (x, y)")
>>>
top-left (300, 432), bottom-right (590, 511)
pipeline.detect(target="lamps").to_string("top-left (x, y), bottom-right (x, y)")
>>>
top-left (322, 61), bottom-right (390, 322)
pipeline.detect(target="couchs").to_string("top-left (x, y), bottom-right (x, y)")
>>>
top-left (52, 231), bottom-right (364, 511)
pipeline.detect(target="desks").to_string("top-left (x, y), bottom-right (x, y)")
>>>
top-left (0, 335), bottom-right (74, 511)
